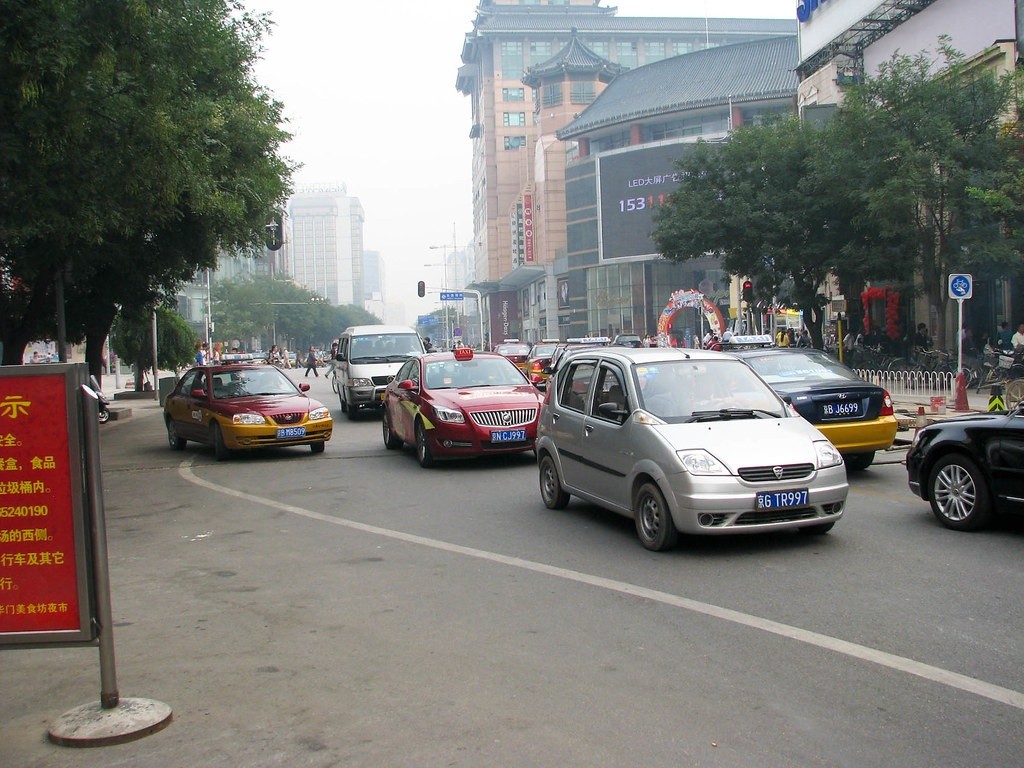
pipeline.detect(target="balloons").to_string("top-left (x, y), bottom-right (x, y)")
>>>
top-left (859, 285), bottom-right (901, 340)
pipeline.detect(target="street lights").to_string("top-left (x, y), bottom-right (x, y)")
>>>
top-left (424, 246), bottom-right (459, 352)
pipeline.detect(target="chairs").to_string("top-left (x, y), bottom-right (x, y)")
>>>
top-left (204, 378), bottom-right (227, 398)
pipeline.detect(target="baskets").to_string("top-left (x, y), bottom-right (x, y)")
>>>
top-left (918, 353), bottom-right (931, 367)
top-left (982, 354), bottom-right (999, 368)
top-left (999, 355), bottom-right (1014, 370)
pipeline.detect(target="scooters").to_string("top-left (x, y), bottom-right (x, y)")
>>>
top-left (90, 375), bottom-right (111, 424)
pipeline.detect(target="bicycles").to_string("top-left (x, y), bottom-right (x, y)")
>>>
top-left (855, 345), bottom-right (908, 381)
top-left (906, 337), bottom-right (1024, 394)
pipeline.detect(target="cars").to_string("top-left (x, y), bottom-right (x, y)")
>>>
top-left (492, 344), bottom-right (531, 370)
top-left (380, 353), bottom-right (545, 468)
top-left (523, 342), bottom-right (619, 394)
top-left (163, 364), bottom-right (333, 461)
top-left (241, 351), bottom-right (331, 368)
top-left (728, 348), bottom-right (898, 472)
top-left (534, 347), bottom-right (849, 550)
top-left (613, 334), bottom-right (644, 348)
top-left (906, 402), bottom-right (1024, 531)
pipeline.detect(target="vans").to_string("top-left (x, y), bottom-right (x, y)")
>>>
top-left (333, 325), bottom-right (427, 419)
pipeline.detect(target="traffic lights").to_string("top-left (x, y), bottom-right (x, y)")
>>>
top-left (743, 281), bottom-right (752, 302)
top-left (743, 313), bottom-right (753, 330)
top-left (418, 281), bottom-right (425, 297)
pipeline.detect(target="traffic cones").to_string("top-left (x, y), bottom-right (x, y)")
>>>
top-left (902, 407), bottom-right (928, 464)
top-left (986, 384), bottom-right (1006, 411)
top-left (951, 372), bottom-right (974, 413)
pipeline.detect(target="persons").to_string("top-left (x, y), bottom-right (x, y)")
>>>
top-left (180, 337), bottom-right (476, 378)
top-left (584, 320), bottom-right (1024, 381)
top-left (30, 351), bottom-right (60, 363)
